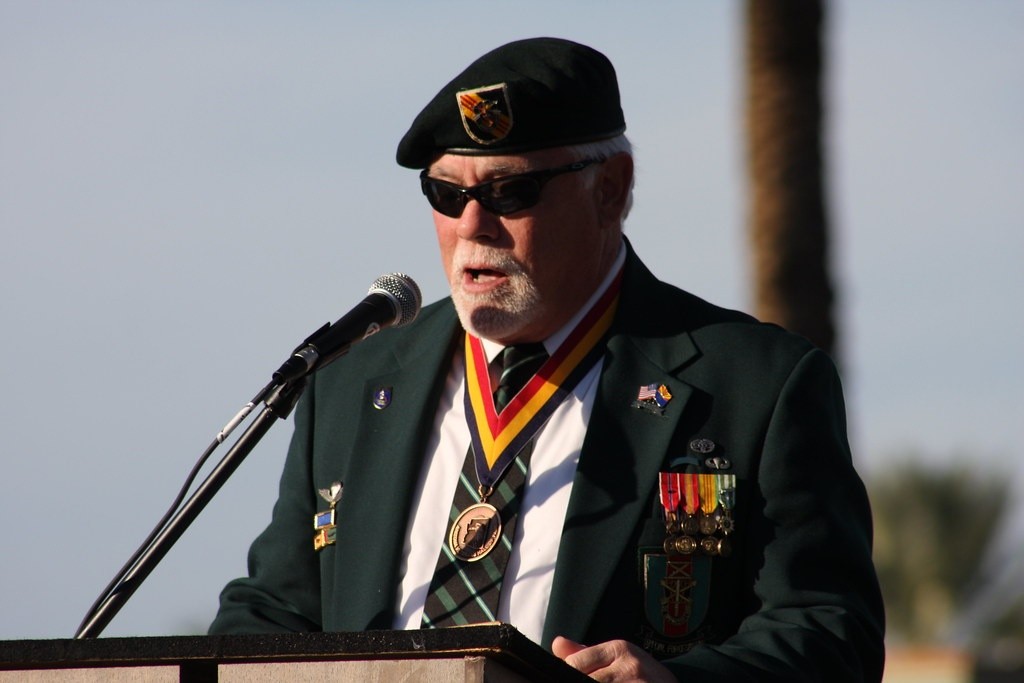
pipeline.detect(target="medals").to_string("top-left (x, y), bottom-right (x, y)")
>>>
top-left (448, 504), bottom-right (502, 562)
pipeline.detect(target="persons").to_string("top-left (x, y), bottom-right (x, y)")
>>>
top-left (207, 38), bottom-right (885, 683)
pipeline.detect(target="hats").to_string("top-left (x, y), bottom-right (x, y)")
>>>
top-left (396, 38), bottom-right (626, 169)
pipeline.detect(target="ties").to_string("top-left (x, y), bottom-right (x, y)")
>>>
top-left (419, 342), bottom-right (552, 629)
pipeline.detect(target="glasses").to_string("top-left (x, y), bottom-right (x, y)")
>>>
top-left (419, 152), bottom-right (617, 219)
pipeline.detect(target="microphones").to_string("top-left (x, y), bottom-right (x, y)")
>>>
top-left (272, 272), bottom-right (423, 385)
